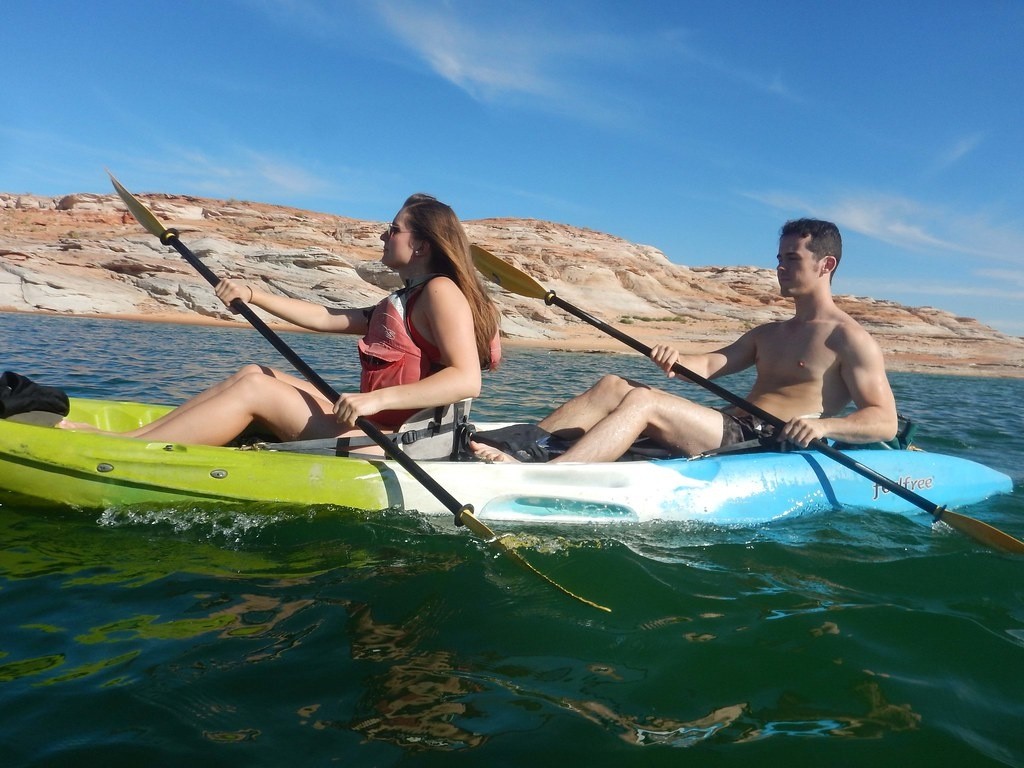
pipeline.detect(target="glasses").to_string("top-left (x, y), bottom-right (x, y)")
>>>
top-left (387, 224), bottom-right (418, 237)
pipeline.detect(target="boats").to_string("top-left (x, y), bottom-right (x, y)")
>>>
top-left (0, 399), bottom-right (1016, 531)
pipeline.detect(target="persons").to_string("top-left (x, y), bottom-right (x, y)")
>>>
top-left (58, 193), bottom-right (501, 447)
top-left (470, 218), bottom-right (899, 462)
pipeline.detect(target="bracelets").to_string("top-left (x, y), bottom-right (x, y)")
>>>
top-left (245, 285), bottom-right (253, 303)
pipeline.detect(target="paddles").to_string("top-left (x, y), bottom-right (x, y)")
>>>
top-left (103, 165), bottom-right (617, 614)
top-left (467, 239), bottom-right (1024, 555)
